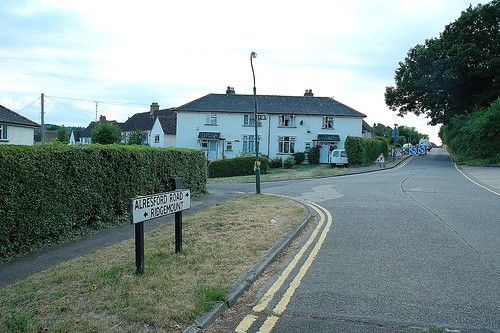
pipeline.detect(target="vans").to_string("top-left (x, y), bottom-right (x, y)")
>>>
top-left (330, 148), bottom-right (348, 166)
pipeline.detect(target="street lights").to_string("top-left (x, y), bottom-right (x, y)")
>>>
top-left (392, 123), bottom-right (398, 161)
top-left (249, 52), bottom-right (263, 193)
